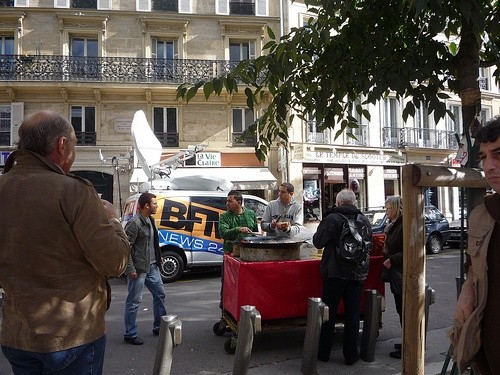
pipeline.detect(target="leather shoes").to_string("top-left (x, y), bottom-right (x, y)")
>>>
top-left (124, 336), bottom-right (144, 345)
top-left (153, 329), bottom-right (160, 336)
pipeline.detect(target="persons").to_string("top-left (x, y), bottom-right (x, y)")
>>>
top-left (312, 188), bottom-right (373, 365)
top-left (0, 109), bottom-right (130, 375)
top-left (218, 189), bottom-right (258, 253)
top-left (124, 192), bottom-right (168, 345)
top-left (383, 196), bottom-right (403, 359)
top-left (447, 115), bottom-right (500, 375)
top-left (260, 182), bottom-right (303, 236)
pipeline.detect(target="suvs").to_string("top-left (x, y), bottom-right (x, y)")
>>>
top-left (362, 205), bottom-right (451, 256)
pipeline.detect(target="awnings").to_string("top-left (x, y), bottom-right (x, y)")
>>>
top-left (127, 165), bottom-right (278, 191)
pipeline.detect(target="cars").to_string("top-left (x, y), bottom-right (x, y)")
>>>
top-left (446, 213), bottom-right (469, 249)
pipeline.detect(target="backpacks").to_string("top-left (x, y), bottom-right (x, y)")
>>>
top-left (334, 213), bottom-right (373, 264)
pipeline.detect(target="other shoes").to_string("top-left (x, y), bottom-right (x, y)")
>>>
top-left (389, 350), bottom-right (401, 359)
top-left (316, 354), bottom-right (330, 362)
top-left (345, 351), bottom-right (359, 365)
top-left (394, 344), bottom-right (402, 349)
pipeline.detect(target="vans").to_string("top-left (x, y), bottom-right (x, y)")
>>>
top-left (121, 190), bottom-right (267, 282)
